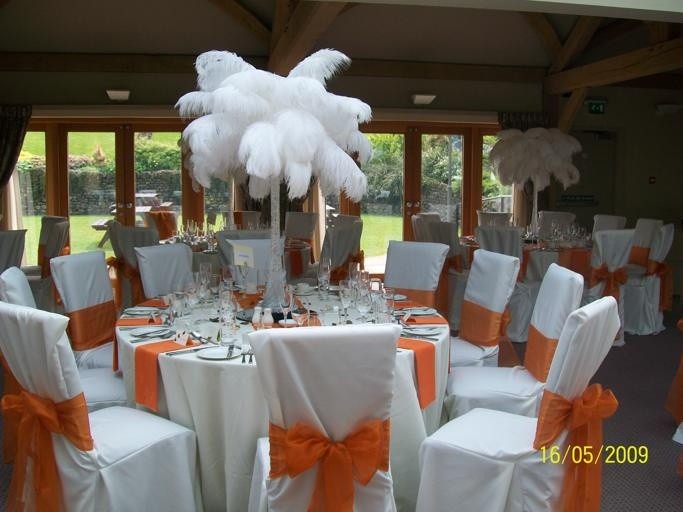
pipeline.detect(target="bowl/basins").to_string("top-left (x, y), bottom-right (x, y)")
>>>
top-left (297, 283), bottom-right (309, 293)
top-left (279, 320), bottom-right (299, 326)
top-left (315, 285), bottom-right (339, 292)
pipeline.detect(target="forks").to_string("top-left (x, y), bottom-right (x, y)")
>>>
top-left (241, 347), bottom-right (247, 364)
top-left (249, 349), bottom-right (253, 363)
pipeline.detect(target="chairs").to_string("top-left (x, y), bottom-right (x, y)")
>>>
top-left (417, 296), bottom-right (622, 511)
top-left (416, 217), bottom-right (470, 331)
top-left (624, 222), bottom-right (675, 336)
top-left (297, 220), bottom-right (363, 287)
top-left (476, 210), bottom-right (513, 230)
top-left (474, 226), bottom-right (541, 343)
top-left (592, 214), bottom-right (627, 238)
top-left (231, 210), bottom-right (260, 233)
top-left (115, 225), bottom-right (160, 306)
top-left (581, 229), bottom-right (635, 348)
top-left (20, 216), bottom-right (69, 277)
top-left (225, 237), bottom-right (287, 286)
top-left (133, 243), bottom-right (192, 301)
top-left (248, 323), bottom-right (402, 512)
top-left (537, 211), bottom-right (577, 238)
top-left (0, 265), bottom-right (129, 412)
top-left (26, 221), bottom-right (69, 313)
top-left (0, 301), bottom-right (206, 511)
top-left (449, 248), bottom-right (520, 368)
top-left (138, 210), bottom-right (179, 241)
top-left (284, 213), bottom-right (318, 245)
top-left (441, 262), bottom-right (584, 422)
top-left (383, 239), bottom-right (449, 309)
top-left (50, 249), bottom-right (119, 367)
top-left (0, 228), bottom-right (27, 274)
top-left (627, 218), bottom-right (663, 275)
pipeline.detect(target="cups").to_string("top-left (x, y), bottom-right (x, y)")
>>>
top-left (340, 280), bottom-right (354, 326)
top-left (279, 294), bottom-right (292, 327)
top-left (173, 292), bottom-right (185, 316)
top-left (200, 263), bottom-right (211, 302)
top-left (349, 263), bottom-right (359, 286)
top-left (356, 291), bottom-right (372, 326)
top-left (166, 293), bottom-right (173, 327)
top-left (318, 278), bottom-right (329, 300)
top-left (209, 274), bottom-right (221, 300)
top-left (317, 264), bottom-right (330, 289)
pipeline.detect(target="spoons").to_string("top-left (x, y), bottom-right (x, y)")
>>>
top-left (191, 332), bottom-right (218, 345)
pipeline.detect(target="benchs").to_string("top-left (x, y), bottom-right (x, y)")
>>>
top-left (160, 200), bottom-right (174, 210)
top-left (91, 217), bottom-right (114, 248)
top-left (110, 208), bottom-right (117, 214)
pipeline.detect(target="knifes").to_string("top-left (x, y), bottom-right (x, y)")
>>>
top-left (227, 345), bottom-right (234, 360)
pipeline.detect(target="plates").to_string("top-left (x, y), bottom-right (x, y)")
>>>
top-left (292, 290), bottom-right (314, 295)
top-left (131, 326), bottom-right (169, 337)
top-left (404, 327), bottom-right (441, 335)
top-left (198, 346), bottom-right (241, 359)
top-left (125, 307), bottom-right (158, 315)
top-left (403, 307), bottom-right (437, 315)
top-left (382, 294), bottom-right (406, 300)
